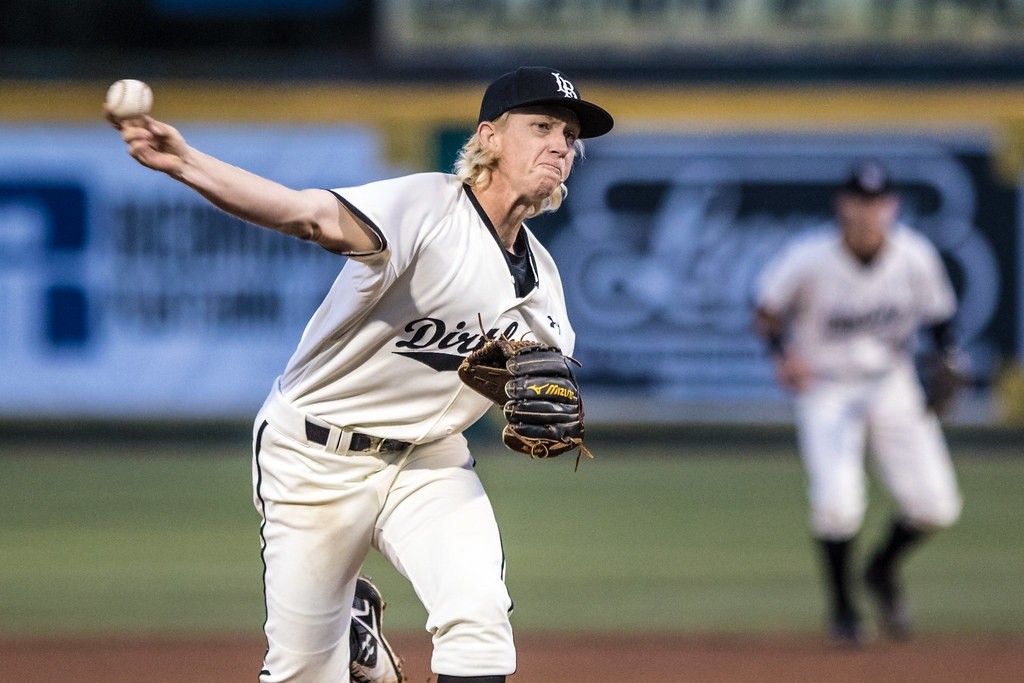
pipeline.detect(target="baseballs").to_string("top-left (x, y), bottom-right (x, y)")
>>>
top-left (105, 78), bottom-right (154, 119)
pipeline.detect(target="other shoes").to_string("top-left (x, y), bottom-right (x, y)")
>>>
top-left (864, 567), bottom-right (910, 640)
top-left (831, 606), bottom-right (860, 643)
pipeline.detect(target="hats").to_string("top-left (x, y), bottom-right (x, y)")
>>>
top-left (477, 66), bottom-right (614, 139)
top-left (836, 160), bottom-right (897, 197)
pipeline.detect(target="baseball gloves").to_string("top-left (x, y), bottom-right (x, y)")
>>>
top-left (457, 339), bottom-right (583, 458)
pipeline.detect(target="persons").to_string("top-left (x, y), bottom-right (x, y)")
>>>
top-left (102, 67), bottom-right (616, 681)
top-left (744, 154), bottom-right (965, 657)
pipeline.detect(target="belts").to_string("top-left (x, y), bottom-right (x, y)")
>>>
top-left (305, 420), bottom-right (411, 456)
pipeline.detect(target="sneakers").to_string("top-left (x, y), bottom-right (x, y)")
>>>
top-left (349, 575), bottom-right (406, 683)
